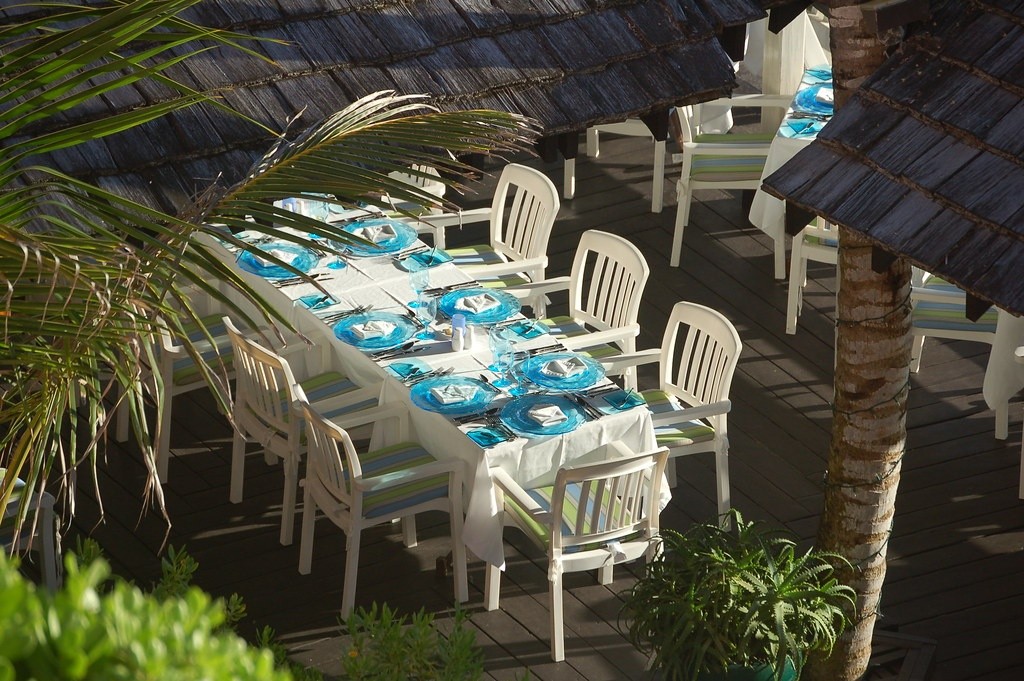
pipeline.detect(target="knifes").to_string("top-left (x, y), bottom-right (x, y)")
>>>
top-left (508, 344), bottom-right (568, 361)
top-left (278, 273), bottom-right (334, 286)
top-left (308, 296), bottom-right (329, 309)
top-left (371, 340), bottom-right (431, 361)
top-left (330, 211), bottom-right (385, 224)
top-left (525, 315), bottom-right (544, 334)
top-left (619, 387), bottom-right (634, 407)
top-left (424, 280), bottom-right (483, 296)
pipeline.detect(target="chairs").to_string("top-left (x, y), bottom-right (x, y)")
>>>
top-left (108, 50), bottom-right (1021, 665)
top-left (0, 467), bottom-right (57, 591)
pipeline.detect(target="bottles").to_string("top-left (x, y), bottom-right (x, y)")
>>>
top-left (452, 328), bottom-right (464, 352)
top-left (465, 328), bottom-right (474, 350)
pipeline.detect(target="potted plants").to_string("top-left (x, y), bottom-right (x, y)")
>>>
top-left (608, 500), bottom-right (863, 681)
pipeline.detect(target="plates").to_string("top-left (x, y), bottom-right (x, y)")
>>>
top-left (523, 353), bottom-right (606, 389)
top-left (410, 376), bottom-right (496, 414)
top-left (440, 288), bottom-right (520, 323)
top-left (467, 427), bottom-right (509, 446)
top-left (796, 83), bottom-right (834, 115)
top-left (501, 395), bottom-right (586, 434)
top-left (236, 243), bottom-right (319, 279)
top-left (333, 310), bottom-right (416, 349)
top-left (338, 219), bottom-right (418, 255)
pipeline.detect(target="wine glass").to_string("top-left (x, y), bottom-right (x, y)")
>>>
top-left (308, 196), bottom-right (328, 238)
top-left (509, 350), bottom-right (530, 396)
top-left (415, 296), bottom-right (437, 340)
top-left (492, 340), bottom-right (514, 388)
top-left (408, 263), bottom-right (429, 308)
top-left (327, 239), bottom-right (347, 269)
top-left (488, 326), bottom-right (509, 371)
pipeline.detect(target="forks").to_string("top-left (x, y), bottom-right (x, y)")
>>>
top-left (798, 123), bottom-right (814, 133)
top-left (325, 304), bottom-right (374, 324)
top-left (403, 365), bottom-right (456, 386)
top-left (224, 235), bottom-right (277, 253)
top-left (452, 408), bottom-right (518, 439)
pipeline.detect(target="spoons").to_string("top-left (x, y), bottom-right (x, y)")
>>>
top-left (480, 374), bottom-right (502, 393)
top-left (437, 308), bottom-right (448, 319)
top-left (517, 372), bottom-right (541, 388)
top-left (394, 244), bottom-right (439, 265)
top-left (564, 382), bottom-right (620, 419)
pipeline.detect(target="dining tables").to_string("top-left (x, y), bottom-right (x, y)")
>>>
top-left (749, 60), bottom-right (838, 278)
top-left (196, 189), bottom-right (673, 589)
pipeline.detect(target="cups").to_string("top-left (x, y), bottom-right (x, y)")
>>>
top-left (452, 314), bottom-right (465, 337)
